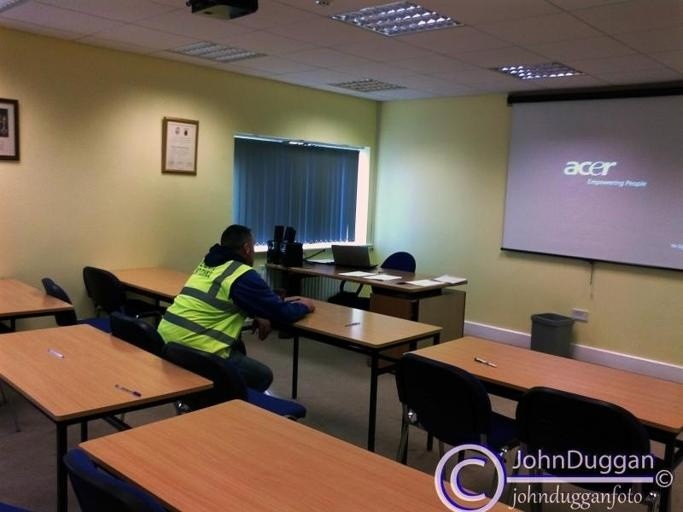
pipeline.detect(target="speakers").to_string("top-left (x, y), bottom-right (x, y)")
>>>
top-left (274, 225), bottom-right (284, 241)
top-left (267, 240), bottom-right (286, 264)
top-left (280, 242), bottom-right (303, 267)
top-left (284, 227), bottom-right (295, 242)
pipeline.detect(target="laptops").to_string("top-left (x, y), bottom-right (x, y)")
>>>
top-left (332, 245), bottom-right (378, 269)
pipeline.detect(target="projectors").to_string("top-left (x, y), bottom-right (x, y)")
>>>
top-left (192, 0), bottom-right (258, 21)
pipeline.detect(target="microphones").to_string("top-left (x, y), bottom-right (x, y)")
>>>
top-left (303, 249), bottom-right (327, 261)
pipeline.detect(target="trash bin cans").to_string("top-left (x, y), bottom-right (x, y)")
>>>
top-left (530, 313), bottom-right (574, 358)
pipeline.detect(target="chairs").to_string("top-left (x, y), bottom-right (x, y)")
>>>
top-left (40, 277), bottom-right (119, 333)
top-left (395, 354), bottom-right (493, 481)
top-left (339, 251), bottom-right (417, 311)
top-left (110, 311), bottom-right (165, 358)
top-left (63, 447), bottom-right (178, 511)
top-left (515, 386), bottom-right (682, 512)
top-left (163, 341), bottom-right (307, 420)
top-left (83, 265), bottom-right (167, 330)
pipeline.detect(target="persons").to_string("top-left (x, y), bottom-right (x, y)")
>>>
top-left (156, 223), bottom-right (318, 395)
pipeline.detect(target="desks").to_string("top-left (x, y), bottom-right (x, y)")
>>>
top-left (0, 276), bottom-right (76, 431)
top-left (265, 259), bottom-right (468, 376)
top-left (78, 398), bottom-right (524, 511)
top-left (265, 293), bottom-right (445, 454)
top-left (0, 322), bottom-right (215, 511)
top-left (105, 264), bottom-right (255, 332)
top-left (400, 336), bottom-right (683, 512)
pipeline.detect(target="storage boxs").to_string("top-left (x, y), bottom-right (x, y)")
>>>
top-left (370, 289), bottom-right (466, 355)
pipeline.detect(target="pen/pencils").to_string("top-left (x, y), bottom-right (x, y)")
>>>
top-left (115, 383), bottom-right (141, 397)
top-left (48, 349), bottom-right (64, 358)
top-left (475, 356), bottom-right (497, 368)
top-left (287, 298), bottom-right (300, 302)
top-left (345, 322), bottom-right (360, 327)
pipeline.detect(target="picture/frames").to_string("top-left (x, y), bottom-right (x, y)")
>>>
top-left (160, 116), bottom-right (199, 176)
top-left (1, 97), bottom-right (20, 162)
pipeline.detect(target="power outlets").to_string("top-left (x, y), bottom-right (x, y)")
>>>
top-left (571, 307), bottom-right (588, 323)
